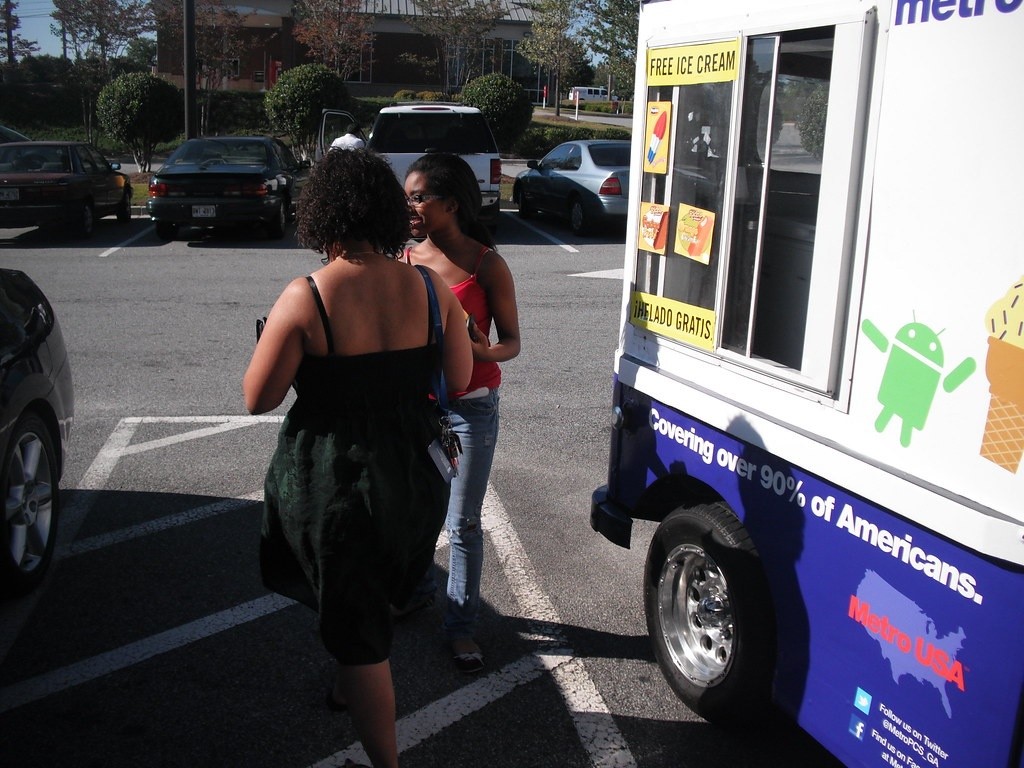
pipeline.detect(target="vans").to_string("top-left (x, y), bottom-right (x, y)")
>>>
top-left (568, 86), bottom-right (618, 103)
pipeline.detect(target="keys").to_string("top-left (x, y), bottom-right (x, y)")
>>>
top-left (438, 414), bottom-right (463, 478)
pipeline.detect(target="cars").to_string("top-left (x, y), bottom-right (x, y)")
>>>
top-left (512, 138), bottom-right (632, 235)
top-left (0, 265), bottom-right (73, 605)
top-left (146, 138), bottom-right (298, 240)
top-left (1, 141), bottom-right (131, 237)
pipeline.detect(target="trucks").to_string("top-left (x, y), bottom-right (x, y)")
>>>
top-left (590, 0), bottom-right (1024, 768)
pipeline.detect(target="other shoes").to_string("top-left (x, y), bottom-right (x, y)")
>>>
top-left (390, 585), bottom-right (438, 618)
top-left (442, 635), bottom-right (486, 673)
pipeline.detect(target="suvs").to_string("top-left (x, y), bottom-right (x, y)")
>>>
top-left (316, 101), bottom-right (501, 227)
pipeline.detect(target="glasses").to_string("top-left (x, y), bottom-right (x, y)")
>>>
top-left (407, 193), bottom-right (449, 206)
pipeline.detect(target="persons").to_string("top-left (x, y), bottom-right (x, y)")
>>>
top-left (326, 123), bottom-right (366, 160)
top-left (388, 152), bottom-right (521, 673)
top-left (242, 145), bottom-right (474, 768)
